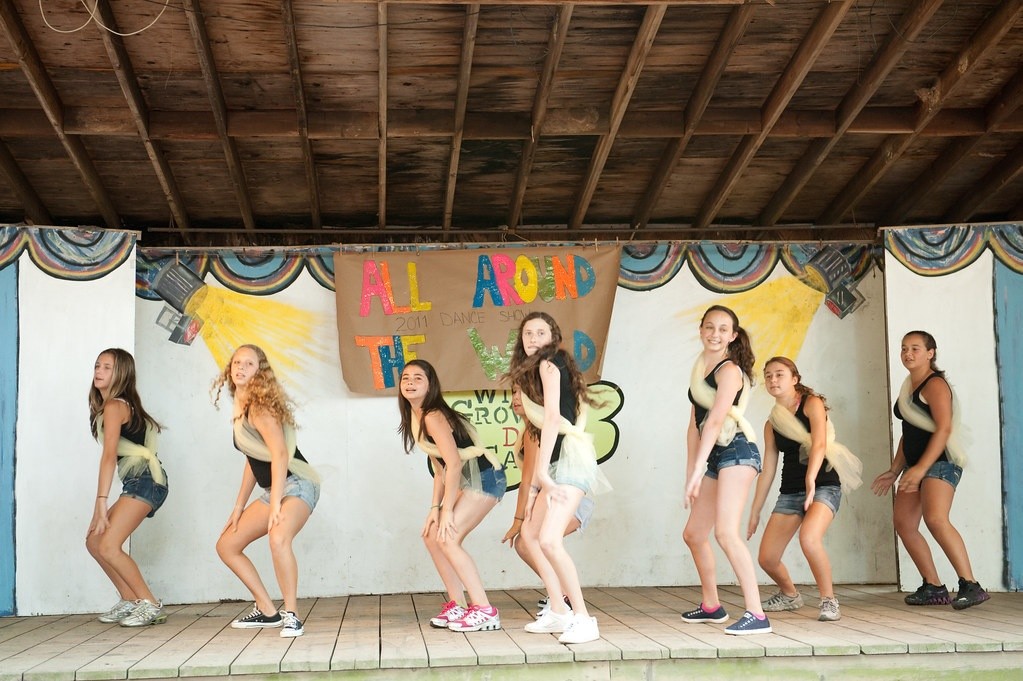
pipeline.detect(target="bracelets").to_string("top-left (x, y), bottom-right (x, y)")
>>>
top-left (514, 517), bottom-right (524, 521)
top-left (99, 496), bottom-right (107, 498)
top-left (530, 485), bottom-right (540, 493)
top-left (431, 505), bottom-right (439, 509)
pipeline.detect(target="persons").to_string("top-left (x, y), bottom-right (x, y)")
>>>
top-left (209, 344), bottom-right (320, 637)
top-left (501, 382), bottom-right (595, 619)
top-left (499, 312), bottom-right (611, 643)
top-left (397, 359), bottom-right (507, 632)
top-left (681, 305), bottom-right (772, 635)
top-left (86, 348), bottom-right (169, 626)
top-left (746, 357), bottom-right (863, 621)
top-left (870, 331), bottom-right (991, 609)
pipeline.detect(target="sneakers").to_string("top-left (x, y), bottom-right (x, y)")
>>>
top-left (680, 603), bottom-right (730, 624)
top-left (430, 600), bottom-right (471, 628)
top-left (559, 617), bottom-right (599, 643)
top-left (524, 609), bottom-right (574, 632)
top-left (535, 595), bottom-right (572, 619)
top-left (904, 577), bottom-right (951, 605)
top-left (817, 598), bottom-right (841, 621)
top-left (951, 577), bottom-right (991, 611)
top-left (761, 590), bottom-right (804, 612)
top-left (724, 611), bottom-right (773, 635)
top-left (447, 605), bottom-right (501, 631)
top-left (230, 607), bottom-right (283, 628)
top-left (97, 599), bottom-right (136, 623)
top-left (119, 599), bottom-right (168, 627)
top-left (279, 610), bottom-right (304, 637)
